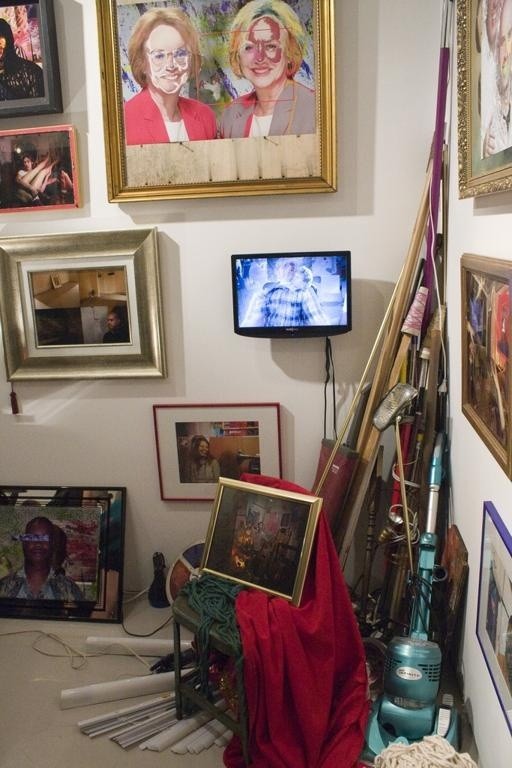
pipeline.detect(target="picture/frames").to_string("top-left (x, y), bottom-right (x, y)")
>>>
top-left (95, 0), bottom-right (339, 204)
top-left (197, 476), bottom-right (326, 609)
top-left (0, 0), bottom-right (64, 119)
top-left (0, 226), bottom-right (169, 384)
top-left (0, 124), bottom-right (81, 214)
top-left (0, 485), bottom-right (128, 624)
top-left (151, 402), bottom-right (283, 502)
top-left (475, 500), bottom-right (512, 738)
top-left (460, 252), bottom-right (512, 480)
top-left (455, 0), bottom-right (512, 201)
top-left (49, 274), bottom-right (63, 289)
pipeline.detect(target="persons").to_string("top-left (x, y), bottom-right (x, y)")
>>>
top-left (0, 517), bottom-right (84, 602)
top-left (483, 0), bottom-right (512, 158)
top-left (217, 1), bottom-right (318, 137)
top-left (189, 434), bottom-right (226, 482)
top-left (98, 306), bottom-right (129, 343)
top-left (117, 4), bottom-right (216, 145)
top-left (13, 151), bottom-right (62, 205)
top-left (0, 17), bottom-right (45, 99)
top-left (58, 165), bottom-right (73, 196)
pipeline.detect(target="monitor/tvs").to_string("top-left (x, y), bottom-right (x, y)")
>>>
top-left (231, 251), bottom-right (352, 338)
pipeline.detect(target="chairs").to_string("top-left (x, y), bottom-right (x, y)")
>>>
top-left (169, 471), bottom-right (324, 765)
